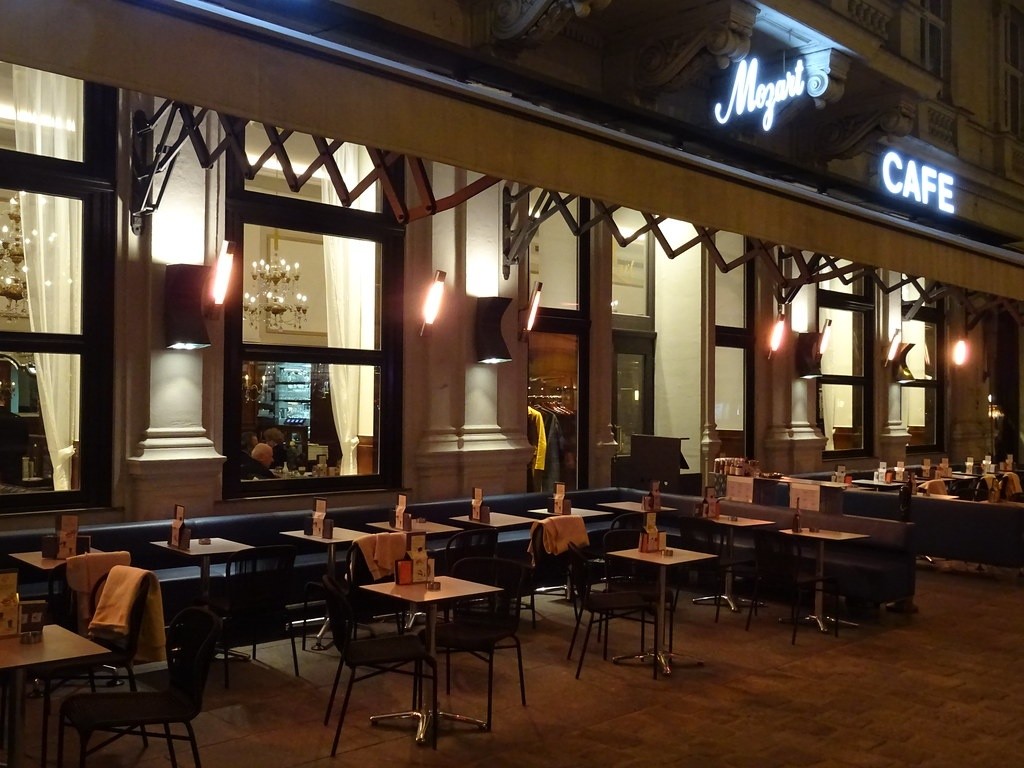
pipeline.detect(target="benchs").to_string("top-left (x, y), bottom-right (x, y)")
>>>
top-left (785, 463), bottom-right (1024, 567)
top-left (224, 486), bottom-right (910, 649)
top-left (620, 487), bottom-right (917, 625)
top-left (0, 517), bottom-right (224, 650)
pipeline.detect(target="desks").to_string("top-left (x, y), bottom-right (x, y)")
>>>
top-left (278, 527), bottom-right (374, 651)
top-left (778, 528), bottom-right (872, 634)
top-left (527, 508), bottom-right (615, 602)
top-left (709, 472), bottom-right (851, 514)
top-left (952, 471), bottom-right (982, 476)
top-left (852, 479), bottom-right (905, 492)
top-left (448, 512), bottom-right (539, 609)
top-left (914, 476), bottom-right (957, 481)
top-left (150, 536), bottom-right (257, 660)
top-left (365, 519), bottom-right (465, 632)
top-left (269, 469), bottom-right (312, 478)
top-left (0, 624), bottom-right (112, 768)
top-left (893, 479), bottom-right (928, 484)
top-left (0, 474), bottom-right (54, 493)
top-left (596, 502), bottom-right (678, 585)
top-left (605, 546), bottom-right (719, 678)
top-left (951, 474), bottom-right (978, 479)
top-left (8, 547), bottom-right (125, 697)
top-left (691, 515), bottom-right (777, 612)
top-left (358, 576), bottom-right (505, 747)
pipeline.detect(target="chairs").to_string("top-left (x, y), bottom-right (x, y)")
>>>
top-left (0, 463), bottom-right (845, 768)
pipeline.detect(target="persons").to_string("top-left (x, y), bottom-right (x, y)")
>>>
top-left (240, 428), bottom-right (301, 479)
top-left (0, 389), bottom-right (29, 486)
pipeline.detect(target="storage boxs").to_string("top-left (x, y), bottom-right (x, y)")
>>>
top-left (274, 362), bottom-right (311, 426)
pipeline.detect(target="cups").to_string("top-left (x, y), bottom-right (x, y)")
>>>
top-left (317, 464), bottom-right (327, 476)
top-left (328, 467), bottom-right (335, 476)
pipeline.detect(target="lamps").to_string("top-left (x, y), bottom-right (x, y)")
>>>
top-left (241, 170), bottom-right (309, 333)
top-left (892, 342), bottom-right (915, 384)
top-left (477, 297), bottom-right (512, 363)
top-left (768, 314), bottom-right (786, 361)
top-left (817, 319), bottom-right (832, 368)
top-left (0, 191), bottom-right (29, 324)
top-left (798, 333), bottom-right (823, 379)
top-left (518, 281), bottom-right (543, 343)
top-left (884, 329), bottom-right (902, 369)
top-left (242, 375), bottom-right (267, 405)
top-left (205, 240), bottom-right (237, 320)
top-left (164, 264), bottom-right (211, 350)
top-left (420, 270), bottom-right (447, 338)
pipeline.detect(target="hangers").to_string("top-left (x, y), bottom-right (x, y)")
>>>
top-left (528, 395), bottom-right (574, 415)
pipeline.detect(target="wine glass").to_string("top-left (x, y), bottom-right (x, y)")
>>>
top-left (298, 466), bottom-right (305, 477)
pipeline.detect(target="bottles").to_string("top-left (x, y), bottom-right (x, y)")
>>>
top-left (282, 462), bottom-right (289, 479)
top-left (906, 469), bottom-right (912, 494)
top-left (714, 458), bottom-right (762, 478)
top-left (898, 486), bottom-right (910, 522)
top-left (792, 497), bottom-right (801, 532)
